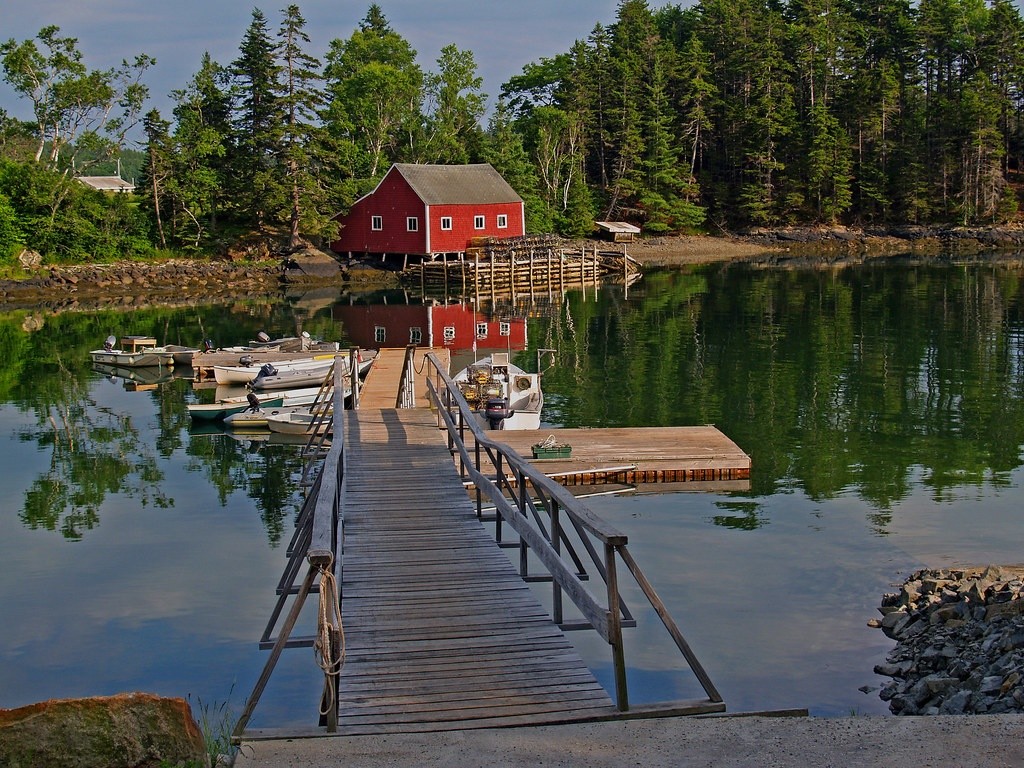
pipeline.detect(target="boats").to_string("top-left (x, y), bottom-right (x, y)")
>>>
top-left (445, 352), bottom-right (544, 431)
top-left (88, 332), bottom-right (377, 437)
